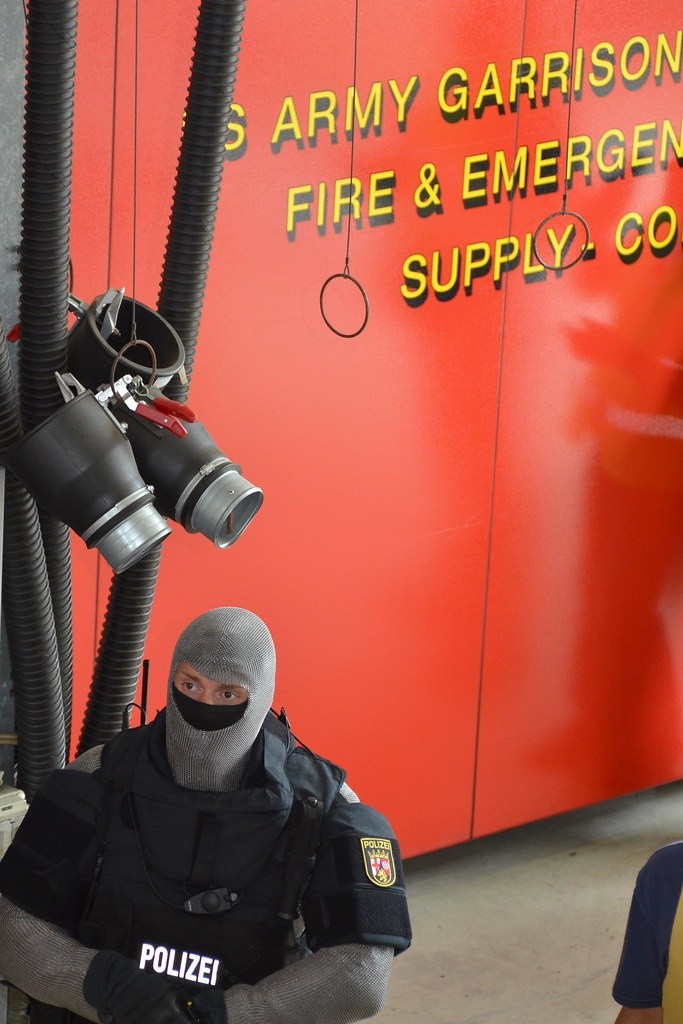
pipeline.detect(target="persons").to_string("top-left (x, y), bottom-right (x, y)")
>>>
top-left (2, 605), bottom-right (413, 1024)
top-left (610, 840), bottom-right (682, 1024)
